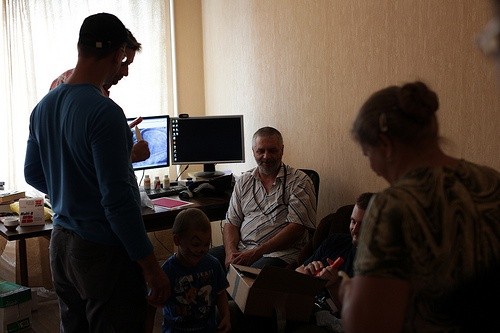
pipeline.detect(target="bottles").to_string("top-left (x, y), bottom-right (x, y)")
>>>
top-left (186, 178), bottom-right (193, 186)
top-left (318, 257), bottom-right (344, 278)
top-left (154, 177), bottom-right (160, 190)
top-left (144, 175), bottom-right (150, 189)
top-left (163, 175), bottom-right (170, 189)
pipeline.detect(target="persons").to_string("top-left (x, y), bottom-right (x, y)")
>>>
top-left (294, 192), bottom-right (385, 317)
top-left (206, 126), bottom-right (320, 333)
top-left (21, 12), bottom-right (171, 333)
top-left (337, 81), bottom-right (500, 333)
top-left (158, 204), bottom-right (232, 333)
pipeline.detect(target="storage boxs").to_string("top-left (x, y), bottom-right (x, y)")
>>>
top-left (0, 281), bottom-right (32, 332)
top-left (225, 263), bottom-right (330, 320)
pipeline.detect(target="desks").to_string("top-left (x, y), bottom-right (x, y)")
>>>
top-left (0, 194), bottom-right (229, 285)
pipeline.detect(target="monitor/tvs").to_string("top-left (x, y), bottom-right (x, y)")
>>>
top-left (126, 115), bottom-right (170, 171)
top-left (169, 114), bottom-right (245, 178)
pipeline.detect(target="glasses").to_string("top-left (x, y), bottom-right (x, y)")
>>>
top-left (121, 49), bottom-right (128, 63)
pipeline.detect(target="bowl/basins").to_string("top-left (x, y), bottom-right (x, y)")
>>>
top-left (4, 218), bottom-right (18, 230)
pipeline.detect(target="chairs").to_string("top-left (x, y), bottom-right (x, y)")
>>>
top-left (285, 169), bottom-right (354, 270)
